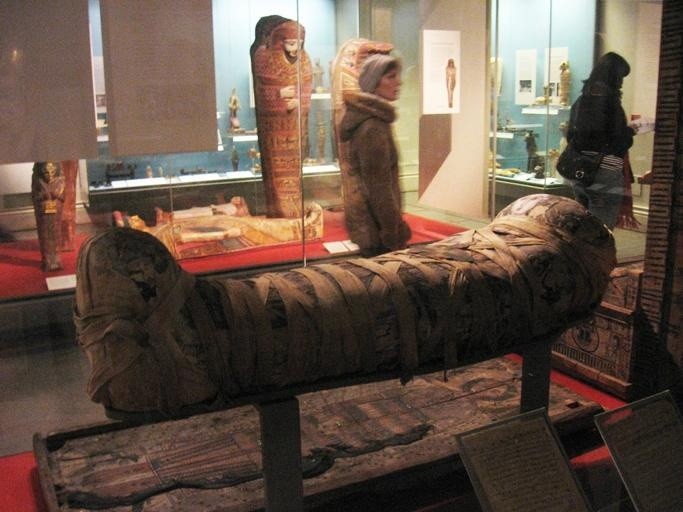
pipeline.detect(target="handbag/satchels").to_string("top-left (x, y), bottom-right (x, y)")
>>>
top-left (555, 145), bottom-right (602, 185)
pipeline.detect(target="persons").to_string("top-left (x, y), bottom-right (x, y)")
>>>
top-left (556, 51), bottom-right (641, 231)
top-left (335, 53), bottom-right (412, 258)
top-left (32, 164), bottom-right (63, 269)
top-left (445, 58), bottom-right (456, 108)
top-left (253, 18), bottom-right (313, 219)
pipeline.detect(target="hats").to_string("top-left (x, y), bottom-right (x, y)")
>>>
top-left (358, 54), bottom-right (395, 94)
